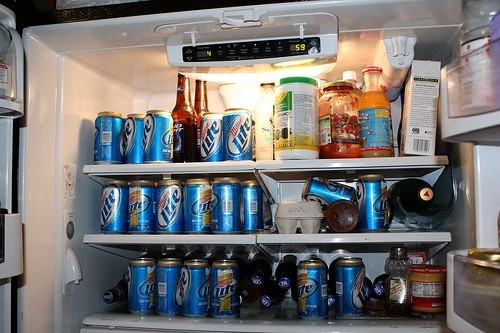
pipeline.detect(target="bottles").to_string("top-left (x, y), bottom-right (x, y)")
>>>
top-left (386, 178), bottom-right (438, 221)
top-left (254, 66), bottom-right (394, 160)
top-left (193, 79), bottom-right (210, 162)
top-left (171, 72), bottom-right (196, 163)
top-left (102, 248), bottom-right (389, 311)
top-left (384, 246), bottom-right (411, 316)
top-left (456, 0), bottom-right (499, 117)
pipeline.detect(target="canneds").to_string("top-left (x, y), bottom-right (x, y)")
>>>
top-left (333, 256), bottom-right (366, 316)
top-left (93, 109), bottom-right (174, 165)
top-left (301, 176), bottom-right (356, 208)
top-left (408, 263), bottom-right (448, 314)
top-left (199, 107), bottom-right (256, 162)
top-left (127, 258), bottom-right (240, 320)
top-left (269, 76), bottom-right (361, 159)
top-left (357, 174), bottom-right (391, 231)
top-left (99, 176), bottom-right (273, 234)
top-left (296, 259), bottom-right (329, 320)
